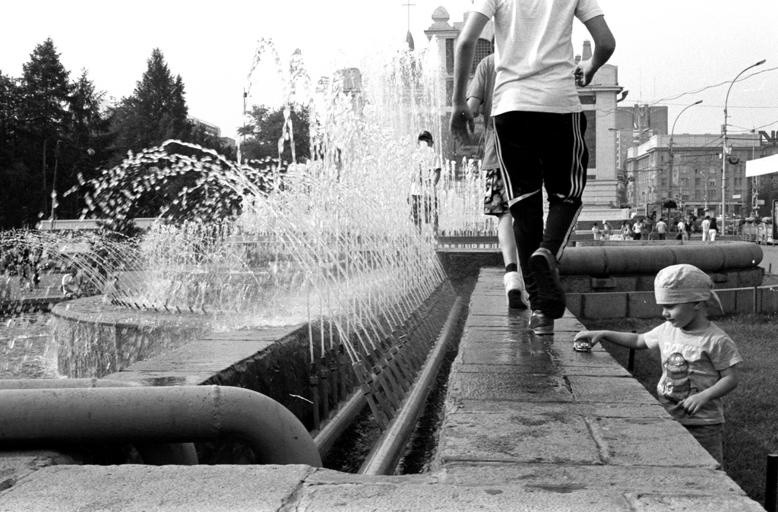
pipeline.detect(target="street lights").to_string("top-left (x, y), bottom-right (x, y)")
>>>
top-left (722, 59), bottom-right (766, 237)
top-left (666, 98), bottom-right (704, 237)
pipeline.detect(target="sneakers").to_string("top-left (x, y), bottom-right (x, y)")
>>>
top-left (531, 249), bottom-right (565, 318)
top-left (505, 271), bottom-right (528, 309)
top-left (529, 310), bottom-right (554, 335)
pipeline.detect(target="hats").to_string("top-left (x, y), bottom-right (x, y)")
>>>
top-left (654, 264), bottom-right (724, 314)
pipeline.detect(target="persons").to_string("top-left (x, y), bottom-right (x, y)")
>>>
top-left (701, 214), bottom-right (710, 241)
top-left (0, 228), bottom-right (111, 299)
top-left (675, 221), bottom-right (688, 241)
top-left (656, 219), bottom-right (668, 239)
top-left (644, 216), bottom-right (654, 242)
top-left (572, 264), bottom-right (742, 472)
top-left (592, 223), bottom-right (600, 240)
top-left (632, 219), bottom-right (643, 239)
top-left (452, 2), bottom-right (615, 333)
top-left (403, 127), bottom-right (442, 239)
top-left (618, 220), bottom-right (632, 241)
top-left (710, 217), bottom-right (716, 241)
top-left (465, 36), bottom-right (529, 308)
top-left (600, 219), bottom-right (612, 240)
